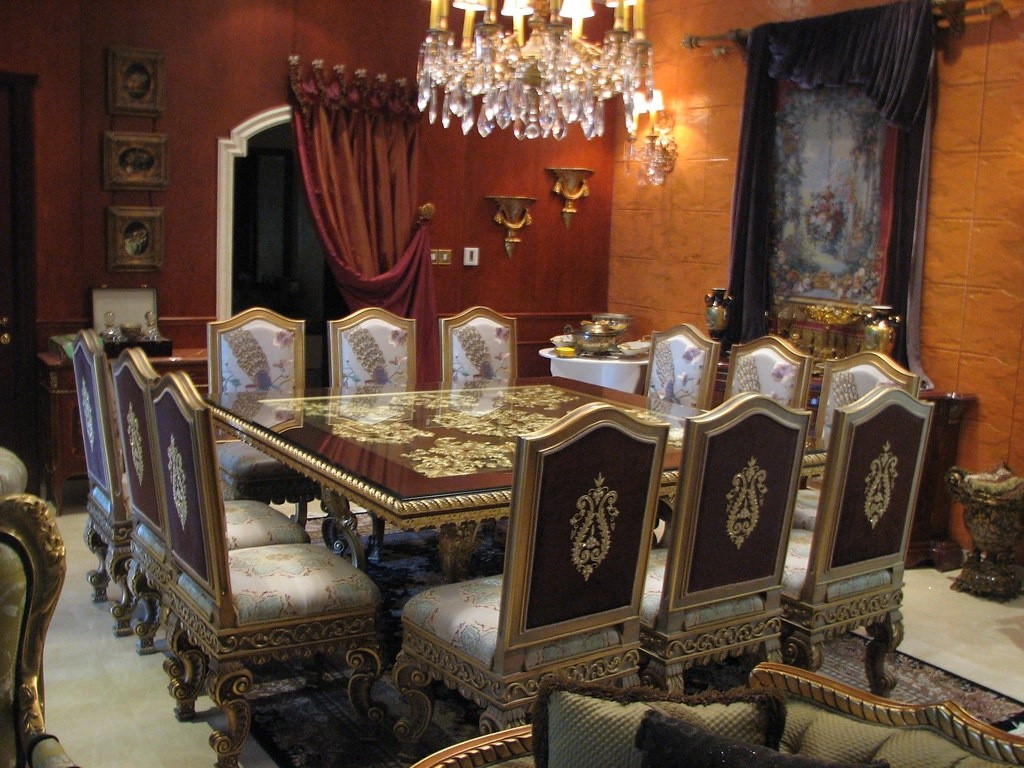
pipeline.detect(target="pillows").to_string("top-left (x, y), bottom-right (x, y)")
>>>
top-left (530, 672), bottom-right (790, 767)
top-left (631, 711), bottom-right (890, 768)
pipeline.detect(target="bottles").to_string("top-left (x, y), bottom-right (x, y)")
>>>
top-left (98, 311), bottom-right (162, 342)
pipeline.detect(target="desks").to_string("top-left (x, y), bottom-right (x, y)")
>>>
top-left (206, 377), bottom-right (710, 596)
top-left (39, 347), bottom-right (210, 518)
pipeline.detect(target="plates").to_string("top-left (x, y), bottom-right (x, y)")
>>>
top-left (550, 334), bottom-right (574, 347)
top-left (618, 342), bottom-right (651, 356)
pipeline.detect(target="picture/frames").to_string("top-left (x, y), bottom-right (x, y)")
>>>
top-left (101, 130), bottom-right (172, 192)
top-left (108, 43), bottom-right (169, 118)
top-left (103, 204), bottom-right (167, 273)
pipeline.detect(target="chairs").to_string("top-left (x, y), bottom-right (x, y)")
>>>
top-left (73, 306), bottom-right (934, 768)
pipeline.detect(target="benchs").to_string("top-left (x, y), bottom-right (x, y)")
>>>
top-left (399, 662), bottom-right (1023, 768)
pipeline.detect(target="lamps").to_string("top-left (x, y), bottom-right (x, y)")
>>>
top-left (415, 0), bottom-right (665, 146)
top-left (618, 86), bottom-right (677, 188)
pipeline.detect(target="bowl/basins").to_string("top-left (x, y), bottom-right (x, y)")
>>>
top-left (592, 313), bottom-right (634, 330)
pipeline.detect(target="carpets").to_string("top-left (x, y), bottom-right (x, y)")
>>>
top-left (226, 621), bottom-right (1023, 768)
